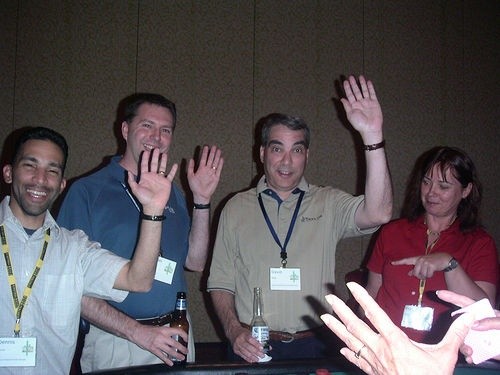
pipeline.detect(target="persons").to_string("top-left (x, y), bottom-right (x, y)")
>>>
top-left (320, 282), bottom-right (500, 375)
top-left (358, 146), bottom-right (499, 365)
top-left (55, 93), bottom-right (224, 373)
top-left (0, 126), bottom-right (179, 375)
top-left (205, 76), bottom-right (394, 364)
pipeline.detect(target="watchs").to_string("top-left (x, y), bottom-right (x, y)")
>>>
top-left (442, 257), bottom-right (459, 272)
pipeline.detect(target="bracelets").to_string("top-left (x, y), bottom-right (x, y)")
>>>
top-left (193, 202), bottom-right (211, 209)
top-left (141, 212), bottom-right (166, 221)
top-left (361, 140), bottom-right (385, 151)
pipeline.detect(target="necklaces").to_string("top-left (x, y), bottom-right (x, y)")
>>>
top-left (430, 230), bottom-right (440, 235)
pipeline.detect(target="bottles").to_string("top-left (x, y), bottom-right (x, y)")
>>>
top-left (167, 291), bottom-right (189, 367)
top-left (250, 288), bottom-right (271, 361)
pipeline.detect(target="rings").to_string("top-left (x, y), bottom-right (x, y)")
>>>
top-left (212, 167), bottom-right (217, 170)
top-left (158, 171), bottom-right (165, 174)
top-left (355, 344), bottom-right (366, 360)
